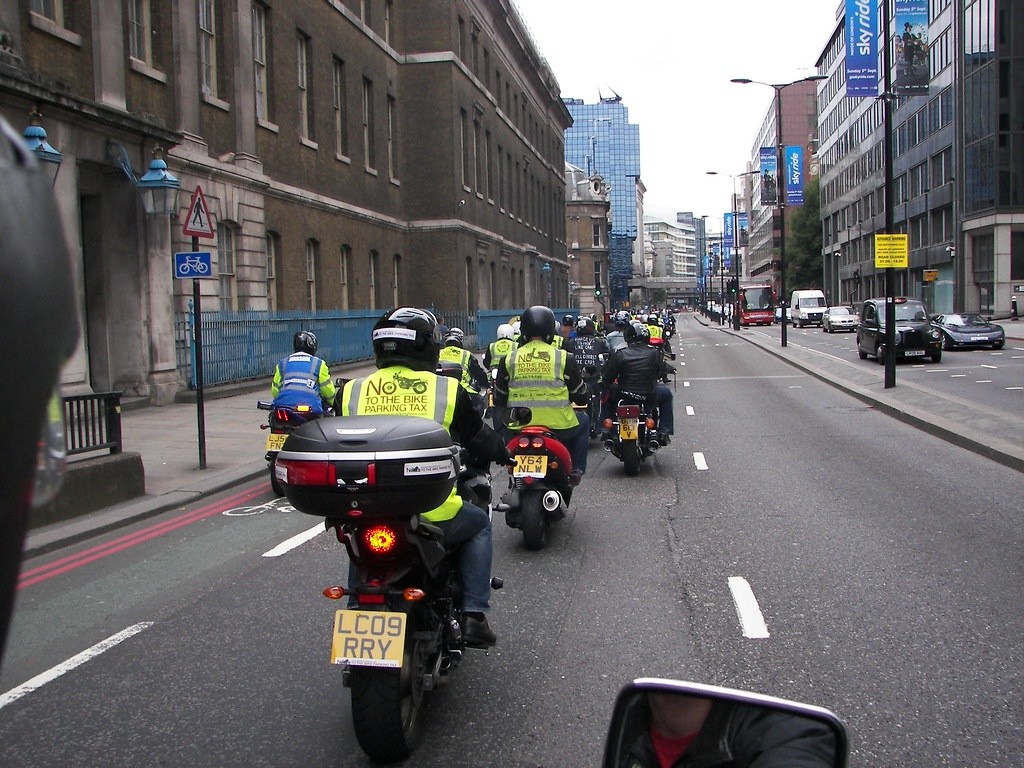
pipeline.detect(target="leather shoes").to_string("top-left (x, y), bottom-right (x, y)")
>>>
top-left (659, 434), bottom-right (671, 446)
top-left (462, 616), bottom-right (497, 646)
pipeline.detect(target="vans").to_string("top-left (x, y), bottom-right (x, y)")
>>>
top-left (791, 290), bottom-right (827, 328)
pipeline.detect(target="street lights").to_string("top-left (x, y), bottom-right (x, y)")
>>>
top-left (731, 76), bottom-right (830, 346)
top-left (706, 171), bottom-right (761, 329)
top-left (702, 215), bottom-right (740, 325)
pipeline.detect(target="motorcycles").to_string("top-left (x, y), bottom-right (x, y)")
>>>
top-left (570, 351), bottom-right (676, 474)
top-left (257, 378), bottom-right (354, 496)
top-left (435, 362), bottom-right (598, 550)
top-left (275, 407), bottom-right (534, 762)
top-left (652, 318), bottom-right (675, 382)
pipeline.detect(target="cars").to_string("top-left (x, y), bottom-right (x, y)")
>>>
top-left (857, 296), bottom-right (943, 366)
top-left (707, 301), bottom-right (733, 321)
top-left (823, 305), bottom-right (861, 332)
top-left (666, 308), bottom-right (679, 314)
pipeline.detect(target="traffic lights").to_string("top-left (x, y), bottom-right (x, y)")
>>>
top-left (731, 281), bottom-right (735, 293)
top-left (595, 281), bottom-right (601, 295)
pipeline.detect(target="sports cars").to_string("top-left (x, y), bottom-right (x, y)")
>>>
top-left (929, 312), bottom-right (1005, 350)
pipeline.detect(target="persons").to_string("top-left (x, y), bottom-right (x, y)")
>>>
top-left (332, 306), bottom-right (516, 646)
top-left (271, 331), bottom-right (337, 422)
top-left (435, 305), bottom-right (678, 485)
top-left (620, 689), bottom-right (837, 768)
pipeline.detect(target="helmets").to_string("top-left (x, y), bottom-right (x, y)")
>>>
top-left (497, 324), bottom-right (513, 341)
top-left (641, 314), bottom-right (649, 323)
top-left (521, 306), bottom-right (555, 339)
top-left (372, 307), bottom-right (438, 373)
top-left (624, 322), bottom-right (650, 345)
top-left (555, 321), bottom-right (561, 335)
top-left (588, 313), bottom-right (597, 321)
top-left (575, 317), bottom-right (595, 336)
top-left (648, 314), bottom-right (658, 325)
top-left (563, 315), bottom-right (573, 327)
top-left (512, 321), bottom-right (520, 335)
top-left (294, 330), bottom-right (317, 355)
top-left (445, 331), bottom-right (463, 348)
top-left (616, 310), bottom-right (632, 325)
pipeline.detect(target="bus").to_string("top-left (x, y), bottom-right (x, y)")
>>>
top-left (739, 284), bottom-right (774, 326)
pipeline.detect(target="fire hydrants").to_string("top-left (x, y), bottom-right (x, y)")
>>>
top-left (1011, 295), bottom-right (1019, 321)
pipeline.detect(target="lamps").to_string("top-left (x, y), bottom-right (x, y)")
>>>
top-left (835, 252), bottom-right (843, 256)
top-left (20, 108), bottom-right (61, 189)
top-left (945, 245), bottom-right (954, 252)
top-left (109, 140), bottom-right (180, 218)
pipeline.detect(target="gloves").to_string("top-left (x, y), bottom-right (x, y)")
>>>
top-left (496, 446), bottom-right (509, 467)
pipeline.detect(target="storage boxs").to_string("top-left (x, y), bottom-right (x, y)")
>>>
top-left (650, 338), bottom-right (666, 347)
top-left (274, 415), bottom-right (459, 516)
top-left (435, 360), bottom-right (463, 381)
